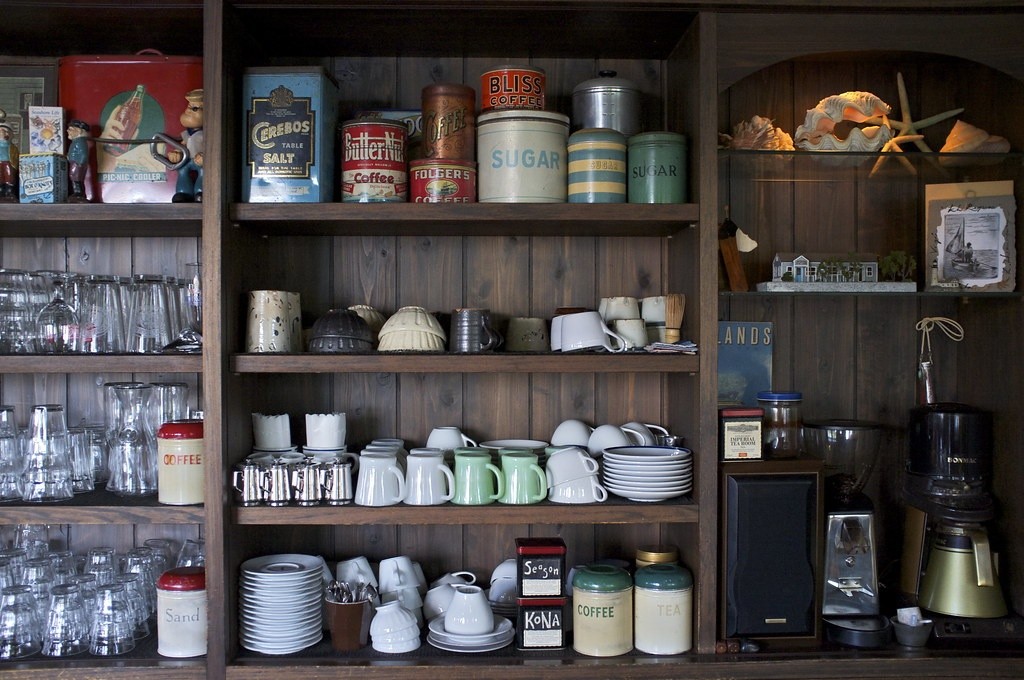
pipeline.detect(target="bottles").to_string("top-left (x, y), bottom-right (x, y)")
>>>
top-left (105, 382), bottom-right (204, 506)
top-left (565, 548), bottom-right (694, 655)
top-left (156, 564), bottom-right (207, 658)
top-left (718, 408), bottom-right (764, 460)
top-left (337, 62), bottom-right (686, 205)
top-left (757, 391), bottom-right (801, 461)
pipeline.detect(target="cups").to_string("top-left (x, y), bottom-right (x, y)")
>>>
top-left (0, 404), bottom-right (107, 503)
top-left (498, 448), bottom-right (533, 469)
top-left (1, 268), bottom-right (55, 353)
top-left (354, 425), bottom-right (505, 505)
top-left (323, 556), bottom-right (518, 655)
top-left (246, 446), bottom-right (298, 459)
top-left (0, 524), bottom-right (204, 659)
top-left (506, 295), bottom-right (672, 350)
top-left (451, 307), bottom-right (501, 356)
top-left (248, 289), bottom-right (301, 353)
top-left (302, 445), bottom-right (359, 475)
top-left (80, 275), bottom-right (193, 353)
top-left (546, 420), bottom-right (667, 508)
top-left (498, 453), bottom-right (547, 503)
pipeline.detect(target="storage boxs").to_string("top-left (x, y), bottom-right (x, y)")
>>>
top-left (17, 104), bottom-right (68, 203)
top-left (241, 65), bottom-right (342, 205)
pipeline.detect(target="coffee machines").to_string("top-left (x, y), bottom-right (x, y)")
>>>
top-left (898, 400), bottom-right (1022, 643)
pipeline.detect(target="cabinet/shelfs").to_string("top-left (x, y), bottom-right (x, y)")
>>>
top-left (0, 0), bottom-right (1024, 680)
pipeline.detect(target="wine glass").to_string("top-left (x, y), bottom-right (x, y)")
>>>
top-left (34, 274), bottom-right (82, 353)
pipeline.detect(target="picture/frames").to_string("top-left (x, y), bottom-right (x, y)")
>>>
top-left (822, 510), bottom-right (883, 620)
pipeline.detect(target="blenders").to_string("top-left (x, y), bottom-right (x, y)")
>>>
top-left (803, 420), bottom-right (890, 648)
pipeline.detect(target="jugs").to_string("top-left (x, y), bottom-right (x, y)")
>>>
top-left (233, 459), bottom-right (351, 507)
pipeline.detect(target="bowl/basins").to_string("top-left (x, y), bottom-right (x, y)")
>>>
top-left (306, 306), bottom-right (445, 353)
top-left (891, 616), bottom-right (933, 646)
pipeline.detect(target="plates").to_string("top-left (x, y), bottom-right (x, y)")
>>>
top-left (479, 439), bottom-right (548, 473)
top-left (242, 555), bottom-right (322, 664)
top-left (601, 447), bottom-right (693, 503)
